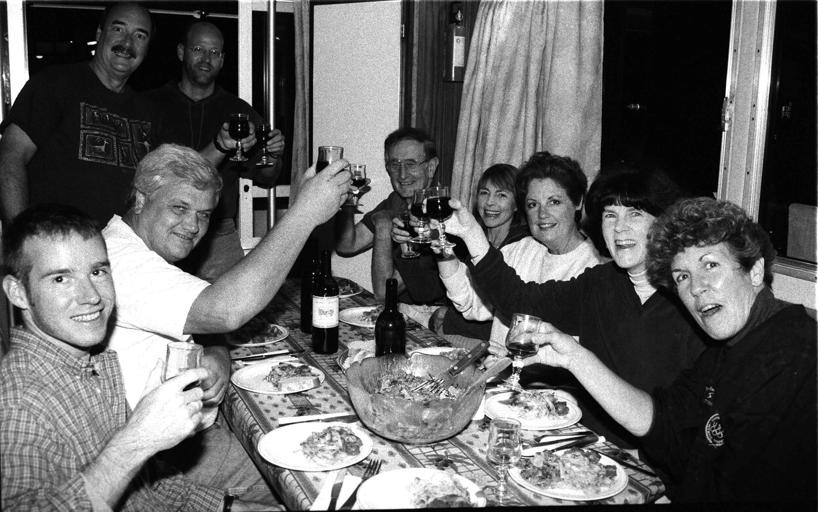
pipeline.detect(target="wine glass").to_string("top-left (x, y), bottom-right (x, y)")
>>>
top-left (254, 120), bottom-right (274, 165)
top-left (497, 312), bottom-right (542, 394)
top-left (343, 164), bottom-right (366, 214)
top-left (228, 113), bottom-right (250, 161)
top-left (409, 189), bottom-right (432, 244)
top-left (426, 187), bottom-right (457, 248)
top-left (316, 146), bottom-right (343, 174)
top-left (398, 209), bottom-right (421, 259)
top-left (486, 417), bottom-right (521, 504)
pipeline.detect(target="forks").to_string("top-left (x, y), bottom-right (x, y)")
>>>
top-left (338, 460), bottom-right (383, 510)
top-left (520, 430), bottom-right (594, 446)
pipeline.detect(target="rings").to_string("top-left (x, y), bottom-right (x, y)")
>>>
top-left (192, 402), bottom-right (200, 412)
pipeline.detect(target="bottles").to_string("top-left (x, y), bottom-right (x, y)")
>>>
top-left (440, 2), bottom-right (467, 78)
top-left (311, 249), bottom-right (339, 354)
top-left (301, 249), bottom-right (321, 333)
top-left (374, 279), bottom-right (406, 356)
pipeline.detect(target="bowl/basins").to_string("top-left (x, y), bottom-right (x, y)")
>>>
top-left (346, 352), bottom-right (487, 444)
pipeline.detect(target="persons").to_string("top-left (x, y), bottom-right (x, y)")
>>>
top-left (1, 0), bottom-right (158, 227)
top-left (146, 17), bottom-right (286, 283)
top-left (335, 126), bottom-right (711, 460)
top-left (512, 195), bottom-right (817, 509)
top-left (99, 141), bottom-right (354, 508)
top-left (1, 202), bottom-right (283, 512)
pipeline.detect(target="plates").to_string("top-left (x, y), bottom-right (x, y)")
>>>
top-left (338, 306), bottom-right (377, 328)
top-left (339, 287), bottom-right (363, 299)
top-left (508, 445), bottom-right (628, 501)
top-left (231, 360), bottom-right (326, 394)
top-left (225, 323), bottom-right (289, 346)
top-left (257, 421), bottom-right (374, 470)
top-left (355, 467), bottom-right (486, 510)
top-left (482, 390), bottom-right (582, 430)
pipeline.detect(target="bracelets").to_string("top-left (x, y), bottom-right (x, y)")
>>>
top-left (213, 132), bottom-right (231, 155)
top-left (223, 494), bottom-right (234, 511)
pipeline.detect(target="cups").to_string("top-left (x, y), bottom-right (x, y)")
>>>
top-left (160, 343), bottom-right (203, 392)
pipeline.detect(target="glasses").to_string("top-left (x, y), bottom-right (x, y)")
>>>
top-left (187, 40), bottom-right (224, 60)
top-left (385, 158), bottom-right (428, 173)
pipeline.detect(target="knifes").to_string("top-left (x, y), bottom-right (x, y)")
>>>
top-left (327, 467), bottom-right (348, 510)
